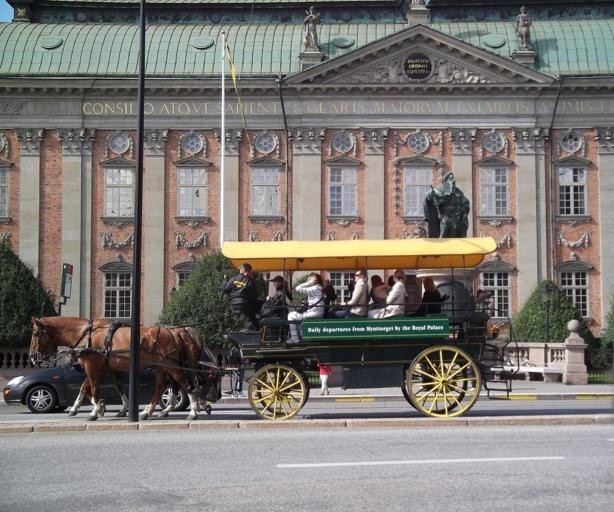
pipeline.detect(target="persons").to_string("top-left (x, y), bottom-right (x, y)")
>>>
top-left (515, 5), bottom-right (532, 49)
top-left (317, 364), bottom-right (333, 394)
top-left (219, 262), bottom-right (441, 347)
top-left (302, 7), bottom-right (321, 51)
top-left (425, 172), bottom-right (472, 236)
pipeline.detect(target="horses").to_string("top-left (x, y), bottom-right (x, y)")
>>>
top-left (27, 314), bottom-right (213, 423)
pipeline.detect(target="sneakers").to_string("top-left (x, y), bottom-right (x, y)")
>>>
top-left (286, 338), bottom-right (300, 343)
top-left (233, 391), bottom-right (330, 397)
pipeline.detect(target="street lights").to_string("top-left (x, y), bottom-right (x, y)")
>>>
top-left (542, 284), bottom-right (554, 343)
top-left (287, 270), bottom-right (294, 301)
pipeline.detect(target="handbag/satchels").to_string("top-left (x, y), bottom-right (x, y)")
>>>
top-left (296, 302), bottom-right (308, 314)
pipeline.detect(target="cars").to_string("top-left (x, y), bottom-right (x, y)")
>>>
top-left (3, 349), bottom-right (222, 414)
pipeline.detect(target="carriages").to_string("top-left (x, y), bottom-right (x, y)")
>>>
top-left (28, 236), bottom-right (520, 421)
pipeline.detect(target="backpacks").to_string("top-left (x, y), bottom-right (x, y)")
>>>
top-left (262, 296), bottom-right (281, 318)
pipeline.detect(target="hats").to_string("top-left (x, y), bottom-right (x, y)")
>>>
top-left (267, 276), bottom-right (283, 283)
